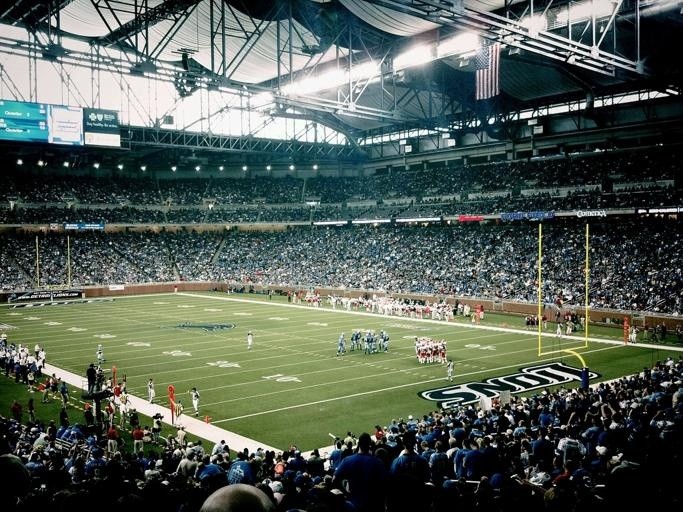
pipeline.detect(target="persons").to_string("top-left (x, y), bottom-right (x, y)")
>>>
top-left (0, 133), bottom-right (682, 512)
